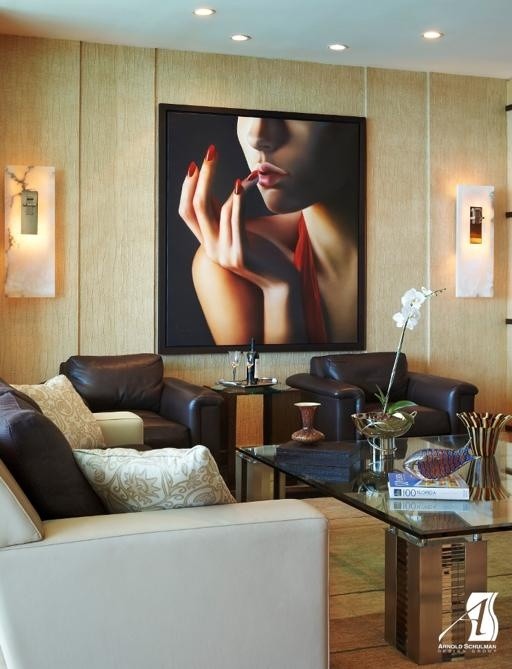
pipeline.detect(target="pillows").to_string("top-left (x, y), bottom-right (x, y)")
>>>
top-left (72, 446), bottom-right (236, 514)
top-left (0, 378), bottom-right (107, 520)
top-left (10, 374), bottom-right (107, 448)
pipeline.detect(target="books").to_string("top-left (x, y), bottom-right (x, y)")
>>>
top-left (386, 472), bottom-right (470, 500)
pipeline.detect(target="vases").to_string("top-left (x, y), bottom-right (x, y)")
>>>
top-left (457, 412), bottom-right (512, 501)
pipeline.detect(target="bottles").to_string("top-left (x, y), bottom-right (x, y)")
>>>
top-left (245, 336), bottom-right (259, 385)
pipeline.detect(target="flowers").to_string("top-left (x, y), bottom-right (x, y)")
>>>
top-left (374, 288), bottom-right (446, 417)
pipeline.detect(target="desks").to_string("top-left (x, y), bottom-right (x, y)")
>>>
top-left (204, 384), bottom-right (308, 502)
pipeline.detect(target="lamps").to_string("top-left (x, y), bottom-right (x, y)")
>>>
top-left (455, 184), bottom-right (495, 298)
top-left (4, 165), bottom-right (56, 298)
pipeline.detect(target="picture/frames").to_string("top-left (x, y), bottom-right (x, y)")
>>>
top-left (354, 412), bottom-right (415, 477)
top-left (156, 102), bottom-right (366, 356)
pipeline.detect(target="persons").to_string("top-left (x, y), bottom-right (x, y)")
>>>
top-left (177, 114), bottom-right (359, 344)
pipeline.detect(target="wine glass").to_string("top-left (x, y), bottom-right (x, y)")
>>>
top-left (227, 351), bottom-right (241, 384)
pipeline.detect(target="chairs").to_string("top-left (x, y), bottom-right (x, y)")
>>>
top-left (286, 352), bottom-right (478, 440)
top-left (40, 354), bottom-right (223, 459)
top-left (0, 412), bottom-right (330, 669)
